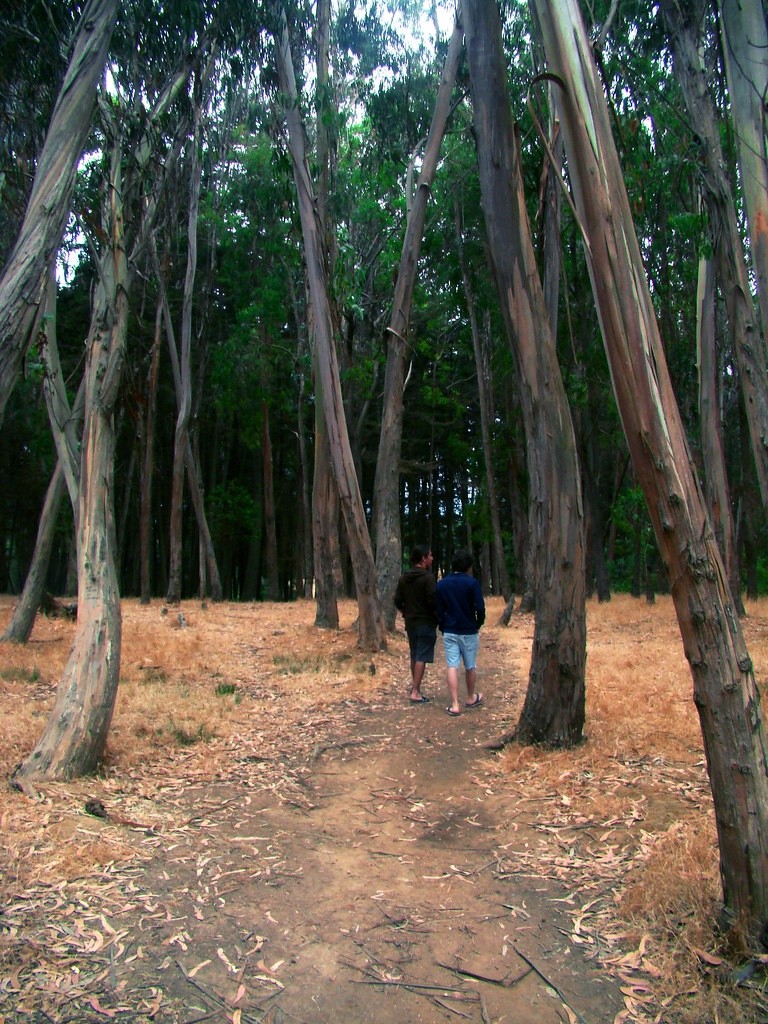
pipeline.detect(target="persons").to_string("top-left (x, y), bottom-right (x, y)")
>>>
top-left (436, 550), bottom-right (486, 716)
top-left (394, 545), bottom-right (438, 703)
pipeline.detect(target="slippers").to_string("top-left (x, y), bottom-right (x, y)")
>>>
top-left (464, 693), bottom-right (484, 708)
top-left (446, 706), bottom-right (459, 716)
top-left (410, 696), bottom-right (430, 704)
